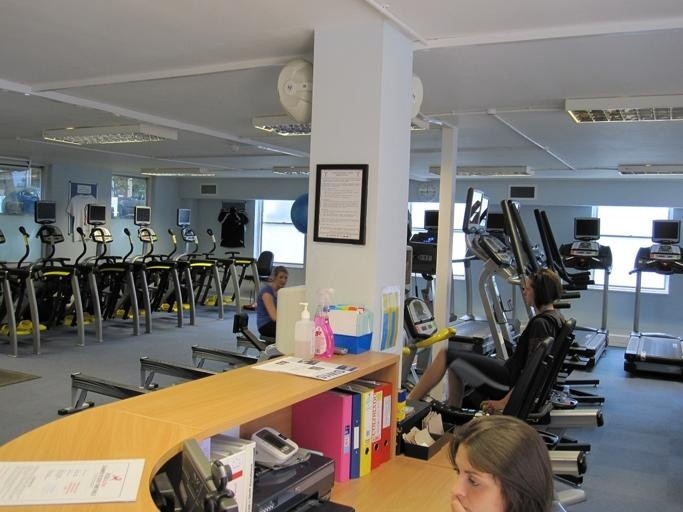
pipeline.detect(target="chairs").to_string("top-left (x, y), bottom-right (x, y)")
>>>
top-left (505, 319), bottom-right (578, 423)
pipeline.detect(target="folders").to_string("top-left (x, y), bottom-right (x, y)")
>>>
top-left (290, 380), bottom-right (393, 483)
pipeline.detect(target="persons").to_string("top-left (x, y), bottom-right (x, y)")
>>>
top-left (257, 266), bottom-right (287, 338)
top-left (449, 414), bottom-right (555, 512)
top-left (407, 268), bottom-right (565, 412)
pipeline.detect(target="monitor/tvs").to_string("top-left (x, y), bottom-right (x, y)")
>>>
top-left (653, 220), bottom-right (680, 243)
top-left (134, 205), bottom-right (151, 226)
top-left (405, 245), bottom-right (413, 291)
top-left (178, 437), bottom-right (239, 512)
top-left (573, 217), bottom-right (600, 239)
top-left (34, 200), bottom-right (56, 224)
top-left (504, 199), bottom-right (521, 237)
top-left (462, 187), bottom-right (490, 233)
top-left (177, 208), bottom-right (191, 226)
top-left (88, 204), bottom-right (106, 225)
top-left (424, 209), bottom-right (439, 230)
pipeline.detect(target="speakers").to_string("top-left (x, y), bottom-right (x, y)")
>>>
top-left (276, 57), bottom-right (313, 124)
top-left (411, 73), bottom-right (424, 120)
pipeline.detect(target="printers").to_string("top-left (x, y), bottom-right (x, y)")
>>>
top-left (252, 453), bottom-right (355, 512)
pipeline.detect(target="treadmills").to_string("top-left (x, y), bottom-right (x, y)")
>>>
top-left (449, 210), bottom-right (520, 355)
top-left (410, 210), bottom-right (457, 322)
top-left (558, 217), bottom-right (612, 370)
top-left (623, 219), bottom-right (683, 377)
